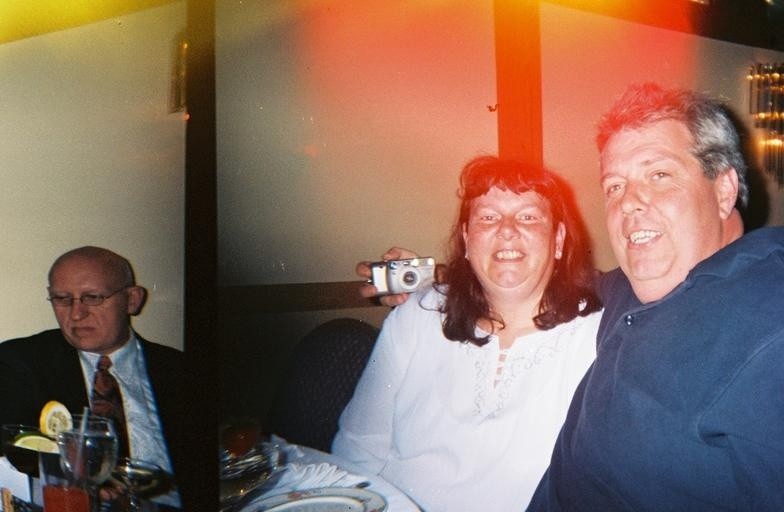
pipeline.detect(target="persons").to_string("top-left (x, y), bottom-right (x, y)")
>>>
top-left (1, 244), bottom-right (185, 510)
top-left (355, 79), bottom-right (783, 512)
top-left (331, 153), bottom-right (604, 512)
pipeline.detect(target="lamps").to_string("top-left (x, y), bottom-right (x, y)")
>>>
top-left (744, 57), bottom-right (784, 196)
top-left (167, 27), bottom-right (186, 116)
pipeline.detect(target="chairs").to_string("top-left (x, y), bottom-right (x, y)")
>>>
top-left (262, 316), bottom-right (382, 454)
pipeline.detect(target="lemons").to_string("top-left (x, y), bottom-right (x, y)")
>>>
top-left (39, 400), bottom-right (73, 439)
top-left (12, 432), bottom-right (60, 454)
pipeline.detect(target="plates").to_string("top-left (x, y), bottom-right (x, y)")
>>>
top-left (240, 484), bottom-right (387, 512)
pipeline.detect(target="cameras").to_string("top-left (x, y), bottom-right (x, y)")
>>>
top-left (367, 257), bottom-right (435, 296)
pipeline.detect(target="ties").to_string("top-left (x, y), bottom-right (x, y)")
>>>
top-left (87, 355), bottom-right (132, 512)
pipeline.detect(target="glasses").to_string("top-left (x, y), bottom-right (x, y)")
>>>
top-left (46, 284), bottom-right (134, 306)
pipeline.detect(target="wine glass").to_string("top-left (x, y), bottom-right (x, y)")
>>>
top-left (0, 414), bottom-right (162, 511)
